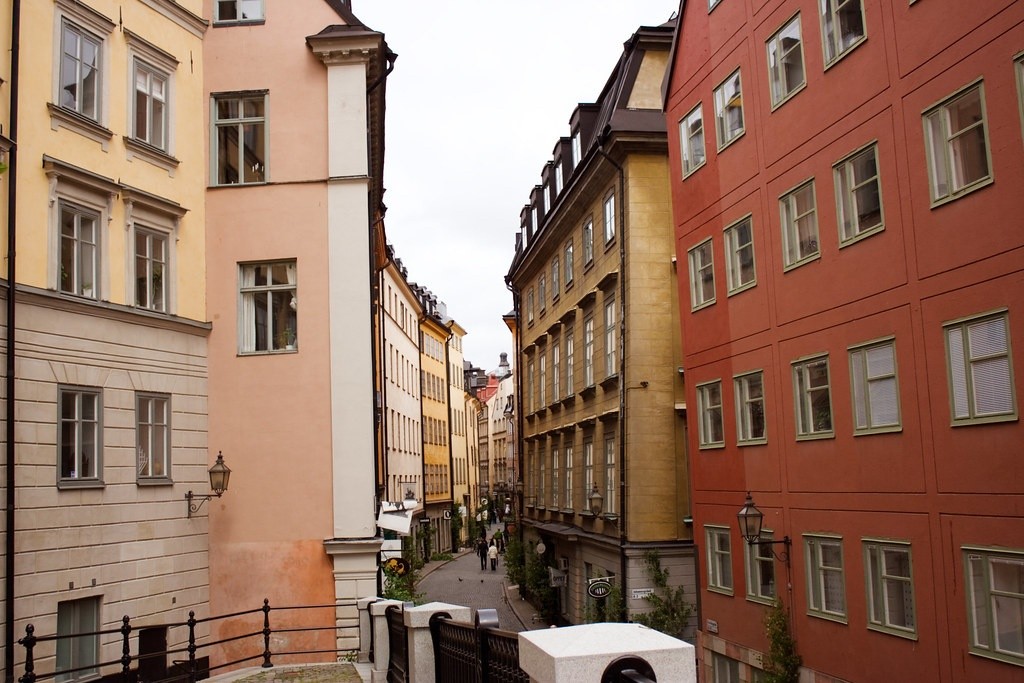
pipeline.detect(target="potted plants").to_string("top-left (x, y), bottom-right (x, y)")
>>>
top-left (282, 327), bottom-right (296, 350)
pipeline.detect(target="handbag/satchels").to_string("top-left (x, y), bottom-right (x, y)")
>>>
top-left (497, 557), bottom-right (498, 566)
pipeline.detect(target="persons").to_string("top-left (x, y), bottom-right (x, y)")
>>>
top-left (489, 529), bottom-right (503, 549)
top-left (480, 526), bottom-right (488, 544)
top-left (477, 539), bottom-right (488, 570)
top-left (490, 507), bottom-right (503, 524)
top-left (487, 542), bottom-right (498, 570)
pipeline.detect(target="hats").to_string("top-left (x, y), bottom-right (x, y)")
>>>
top-left (497, 527), bottom-right (500, 529)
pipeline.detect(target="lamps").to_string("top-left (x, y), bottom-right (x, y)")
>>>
top-left (736, 490), bottom-right (792, 569)
top-left (587, 481), bottom-right (621, 533)
top-left (185, 450), bottom-right (233, 519)
top-left (514, 477), bottom-right (536, 498)
